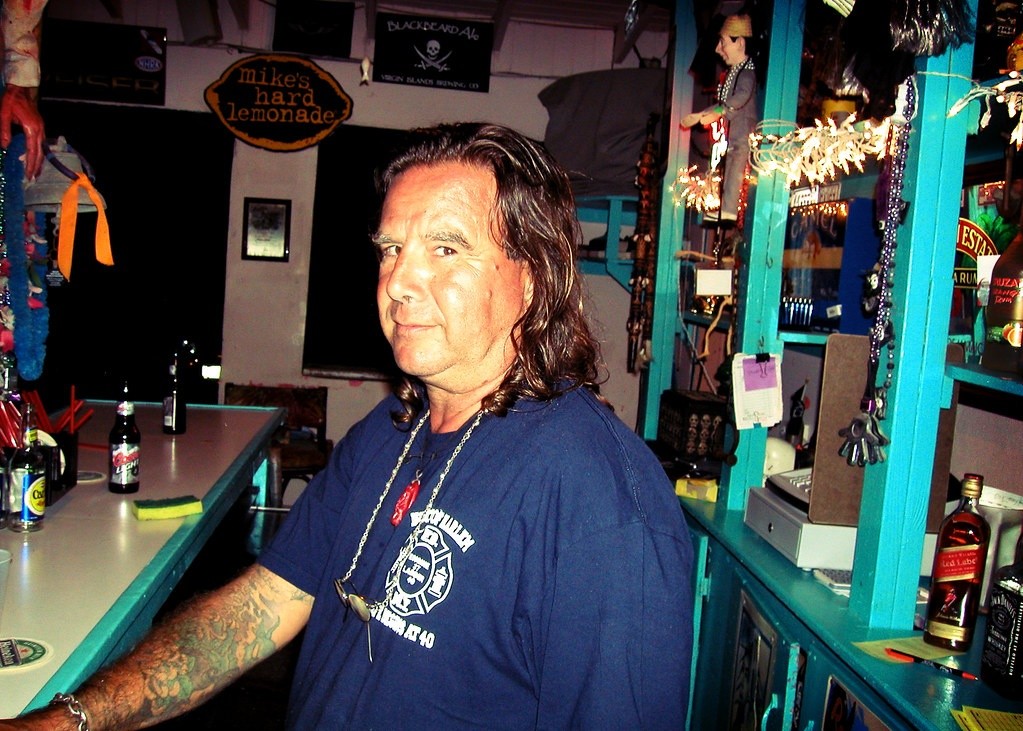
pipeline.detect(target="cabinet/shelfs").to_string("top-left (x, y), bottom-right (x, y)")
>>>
top-left (636, 0), bottom-right (1022, 731)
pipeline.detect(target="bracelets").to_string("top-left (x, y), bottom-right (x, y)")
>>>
top-left (50, 691), bottom-right (90, 731)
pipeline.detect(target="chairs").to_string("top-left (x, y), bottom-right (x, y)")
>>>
top-left (224, 381), bottom-right (331, 504)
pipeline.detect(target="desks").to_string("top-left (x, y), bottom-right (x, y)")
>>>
top-left (1, 398), bottom-right (288, 720)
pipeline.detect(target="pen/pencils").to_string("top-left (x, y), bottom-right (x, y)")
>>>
top-left (884, 647), bottom-right (980, 682)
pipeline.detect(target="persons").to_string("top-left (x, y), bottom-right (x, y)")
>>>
top-left (0, 118), bottom-right (696, 731)
top-left (679, 14), bottom-right (754, 222)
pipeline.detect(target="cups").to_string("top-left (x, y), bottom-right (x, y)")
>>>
top-left (0, 549), bottom-right (13, 617)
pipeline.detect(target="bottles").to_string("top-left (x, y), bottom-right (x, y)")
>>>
top-left (786, 400), bottom-right (804, 448)
top-left (0, 444), bottom-right (11, 530)
top-left (924, 473), bottom-right (991, 651)
top-left (0, 348), bottom-right (16, 401)
top-left (981, 528), bottom-right (1023, 700)
top-left (9, 402), bottom-right (45, 532)
top-left (984, 221), bottom-right (1023, 377)
top-left (109, 381), bottom-right (140, 493)
top-left (163, 353), bottom-right (187, 435)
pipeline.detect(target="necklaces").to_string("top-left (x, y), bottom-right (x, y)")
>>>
top-left (333, 399), bottom-right (483, 612)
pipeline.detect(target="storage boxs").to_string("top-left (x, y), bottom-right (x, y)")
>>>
top-left (2, 429), bottom-right (79, 507)
top-left (746, 486), bottom-right (939, 577)
top-left (658, 388), bottom-right (728, 457)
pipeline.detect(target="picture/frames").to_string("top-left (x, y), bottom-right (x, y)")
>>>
top-left (242, 197), bottom-right (293, 262)
